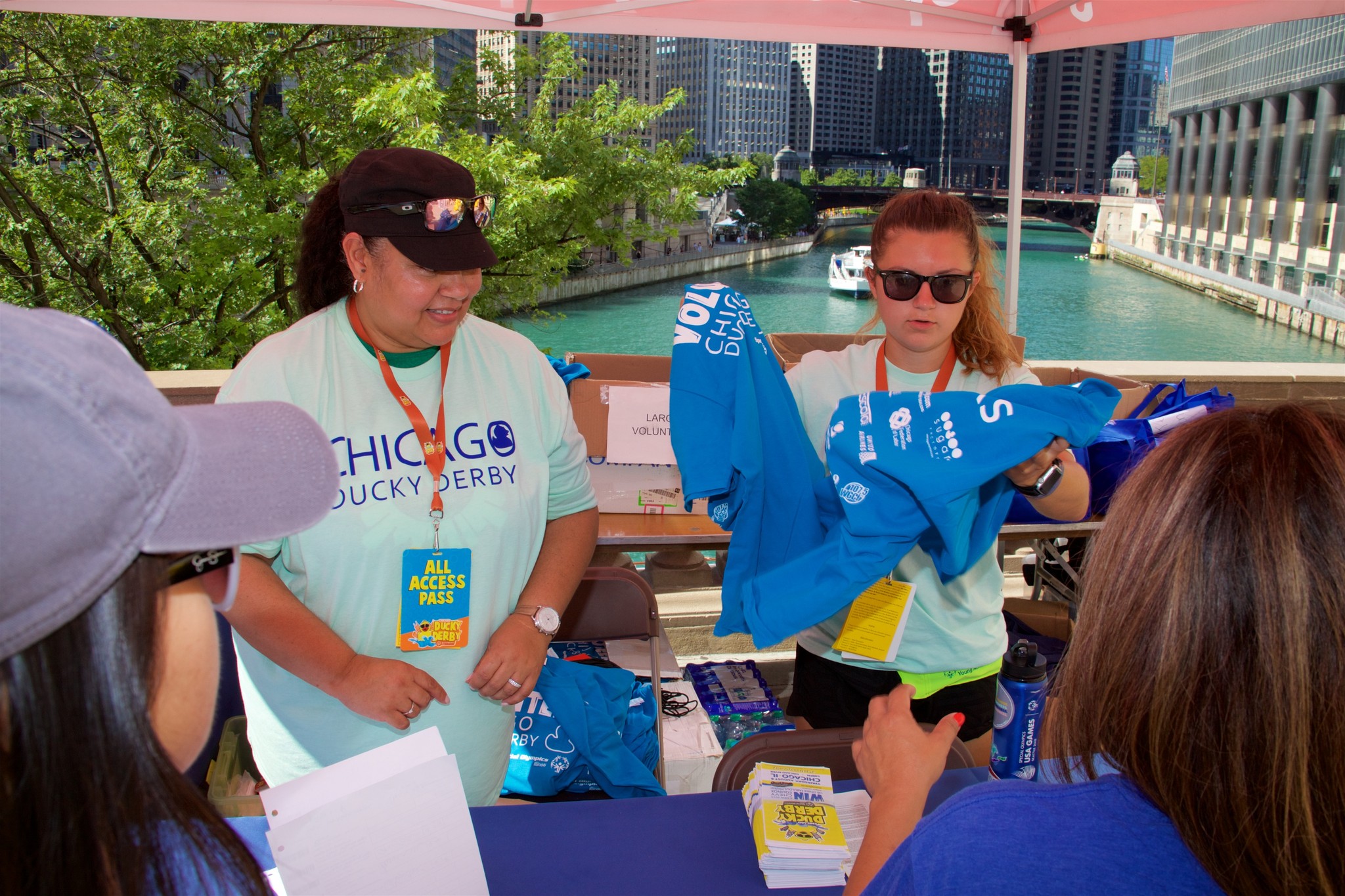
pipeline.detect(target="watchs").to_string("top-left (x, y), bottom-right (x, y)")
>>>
top-left (510, 605), bottom-right (560, 638)
top-left (1010, 456), bottom-right (1064, 499)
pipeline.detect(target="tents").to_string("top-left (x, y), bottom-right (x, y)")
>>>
top-left (0, 0), bottom-right (1345, 334)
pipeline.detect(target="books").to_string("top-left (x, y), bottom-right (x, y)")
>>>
top-left (740, 760), bottom-right (852, 891)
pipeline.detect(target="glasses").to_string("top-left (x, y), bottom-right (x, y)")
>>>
top-left (867, 264), bottom-right (974, 304)
top-left (347, 193), bottom-right (497, 233)
top-left (155, 543), bottom-right (242, 612)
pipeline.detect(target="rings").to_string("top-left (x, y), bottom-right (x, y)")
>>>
top-left (508, 679), bottom-right (521, 688)
top-left (403, 700), bottom-right (414, 714)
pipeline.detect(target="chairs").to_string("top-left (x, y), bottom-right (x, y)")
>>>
top-left (711, 723), bottom-right (977, 794)
top-left (552, 566), bottom-right (666, 791)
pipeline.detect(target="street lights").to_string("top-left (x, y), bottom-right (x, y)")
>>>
top-left (1075, 168), bottom-right (1082, 194)
top-left (992, 166), bottom-right (1000, 189)
top-left (1099, 178), bottom-right (1108, 195)
top-left (1042, 178), bottom-right (1052, 192)
top-left (1050, 177), bottom-right (1061, 193)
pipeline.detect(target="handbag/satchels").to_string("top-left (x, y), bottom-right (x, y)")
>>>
top-left (1004, 377), bottom-right (1236, 524)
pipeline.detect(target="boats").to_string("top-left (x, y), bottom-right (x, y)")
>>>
top-left (827, 246), bottom-right (873, 300)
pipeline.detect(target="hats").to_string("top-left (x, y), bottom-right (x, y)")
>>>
top-left (0, 303), bottom-right (341, 662)
top-left (338, 147), bottom-right (500, 271)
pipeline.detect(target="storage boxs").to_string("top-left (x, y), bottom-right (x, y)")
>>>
top-left (567, 334), bottom-right (1157, 515)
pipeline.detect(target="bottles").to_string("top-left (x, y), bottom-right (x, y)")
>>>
top-left (682, 659), bottom-right (794, 755)
top-left (987, 638), bottom-right (1048, 782)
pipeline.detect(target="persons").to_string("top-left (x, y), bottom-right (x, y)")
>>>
top-left (0, 300), bottom-right (340, 896)
top-left (844, 403), bottom-right (1345, 896)
top-left (209, 148), bottom-right (597, 805)
top-left (679, 190), bottom-right (1091, 766)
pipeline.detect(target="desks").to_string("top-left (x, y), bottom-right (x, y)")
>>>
top-left (132, 751), bottom-right (1123, 896)
top-left (596, 511), bottom-right (1103, 611)
